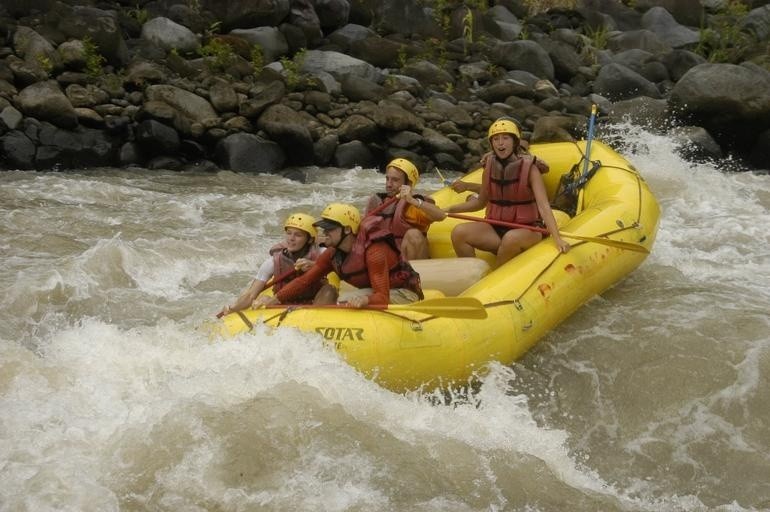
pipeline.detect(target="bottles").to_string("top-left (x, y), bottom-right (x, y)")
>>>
top-left (312, 219), bottom-right (339, 230)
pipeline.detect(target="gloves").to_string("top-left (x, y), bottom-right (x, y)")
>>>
top-left (219, 136), bottom-right (663, 400)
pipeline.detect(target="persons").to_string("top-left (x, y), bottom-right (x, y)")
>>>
top-left (362, 157), bottom-right (449, 262)
top-left (441, 121), bottom-right (571, 266)
top-left (224, 212), bottom-right (337, 318)
top-left (451, 115), bottom-right (552, 205)
top-left (251, 201), bottom-right (425, 311)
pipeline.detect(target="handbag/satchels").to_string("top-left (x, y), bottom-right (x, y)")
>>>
top-left (417, 198), bottom-right (423, 209)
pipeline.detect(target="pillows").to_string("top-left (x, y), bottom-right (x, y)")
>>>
top-left (488, 120), bottom-right (520, 141)
top-left (386, 158), bottom-right (419, 189)
top-left (320, 203), bottom-right (361, 235)
top-left (497, 117), bottom-right (521, 133)
top-left (284, 213), bottom-right (318, 238)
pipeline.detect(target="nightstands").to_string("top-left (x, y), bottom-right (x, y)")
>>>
top-left (444, 212), bottom-right (652, 254)
top-left (435, 166), bottom-right (453, 186)
top-left (259, 296), bottom-right (487, 319)
top-left (576, 104), bottom-right (596, 217)
